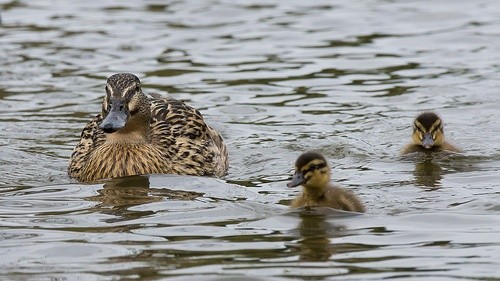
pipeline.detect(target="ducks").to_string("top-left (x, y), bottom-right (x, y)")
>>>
top-left (287, 151), bottom-right (366, 214)
top-left (66, 73), bottom-right (229, 180)
top-left (402, 112), bottom-right (463, 154)
